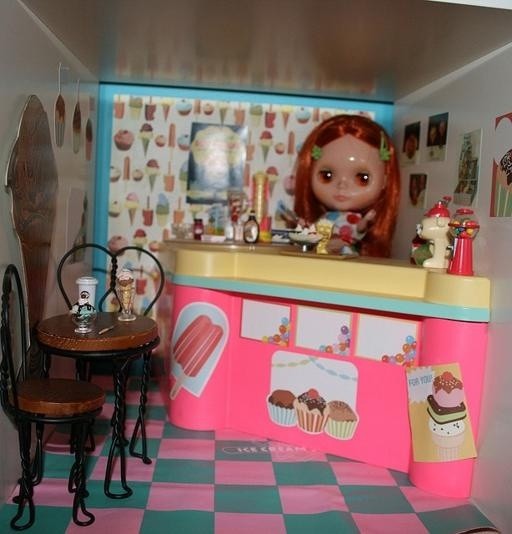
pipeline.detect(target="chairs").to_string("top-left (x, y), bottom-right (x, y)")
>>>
top-left (30, 244), bottom-right (165, 472)
top-left (0, 263), bottom-right (109, 528)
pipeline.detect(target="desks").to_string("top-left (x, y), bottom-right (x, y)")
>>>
top-left (30, 309), bottom-right (162, 499)
